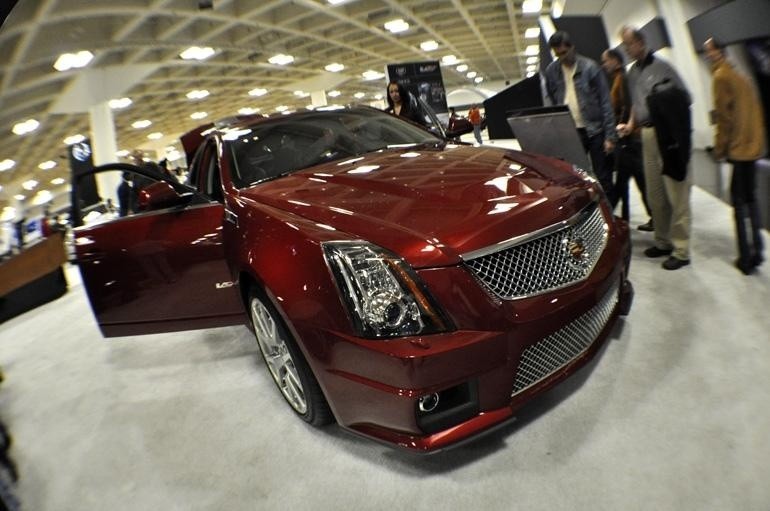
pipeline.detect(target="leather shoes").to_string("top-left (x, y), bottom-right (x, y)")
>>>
top-left (636, 218), bottom-right (655, 231)
top-left (643, 245), bottom-right (672, 257)
top-left (663, 254), bottom-right (692, 270)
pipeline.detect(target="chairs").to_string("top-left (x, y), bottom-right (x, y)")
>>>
top-left (238, 154), bottom-right (267, 182)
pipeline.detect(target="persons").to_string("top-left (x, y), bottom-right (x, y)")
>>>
top-left (158, 158), bottom-right (177, 184)
top-left (427, 123), bottom-right (439, 134)
top-left (118, 173), bottom-right (133, 215)
top-left (51, 217), bottom-right (67, 245)
top-left (543, 29), bottom-right (623, 206)
top-left (173, 167), bottom-right (190, 182)
top-left (448, 107), bottom-right (461, 144)
top-left (701, 35), bottom-right (765, 275)
top-left (41, 211), bottom-right (51, 237)
top-left (619, 25), bottom-right (694, 271)
top-left (597, 49), bottom-right (661, 234)
top-left (380, 81), bottom-right (429, 131)
top-left (468, 103), bottom-right (483, 146)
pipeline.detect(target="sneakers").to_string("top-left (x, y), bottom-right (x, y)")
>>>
top-left (734, 253), bottom-right (764, 276)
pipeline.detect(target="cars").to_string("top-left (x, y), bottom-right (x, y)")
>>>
top-left (69, 101), bottom-right (632, 459)
